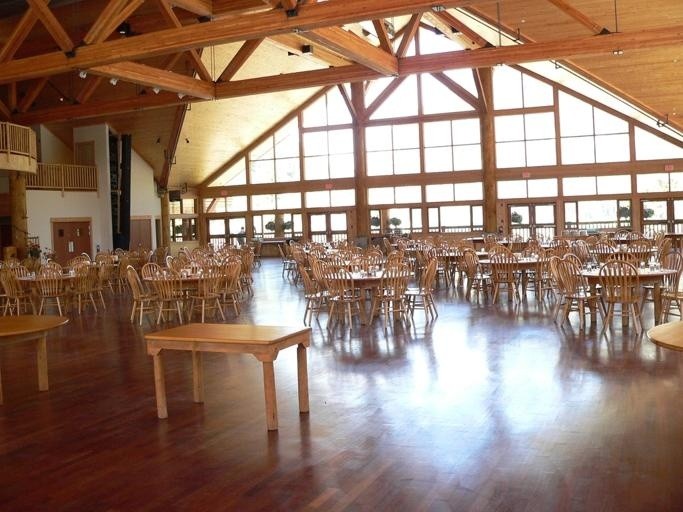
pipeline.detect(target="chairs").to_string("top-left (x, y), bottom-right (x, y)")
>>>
top-left (0, 234), bottom-right (263, 327)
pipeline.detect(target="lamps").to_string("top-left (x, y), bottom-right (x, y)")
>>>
top-left (152, 87), bottom-right (161, 94)
top-left (176, 93), bottom-right (185, 99)
top-left (79, 71), bottom-right (88, 79)
top-left (109, 78), bottom-right (118, 85)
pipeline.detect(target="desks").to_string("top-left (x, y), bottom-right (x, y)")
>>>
top-left (644, 320), bottom-right (683, 353)
top-left (0, 315), bottom-right (70, 406)
top-left (143, 323), bottom-right (313, 432)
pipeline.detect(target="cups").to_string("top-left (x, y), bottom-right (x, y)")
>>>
top-left (603, 241), bottom-right (646, 251)
top-left (1, 247), bottom-right (248, 283)
top-left (305, 242), bottom-right (400, 277)
top-left (404, 237), bottom-right (581, 260)
top-left (585, 257), bottom-right (662, 275)
